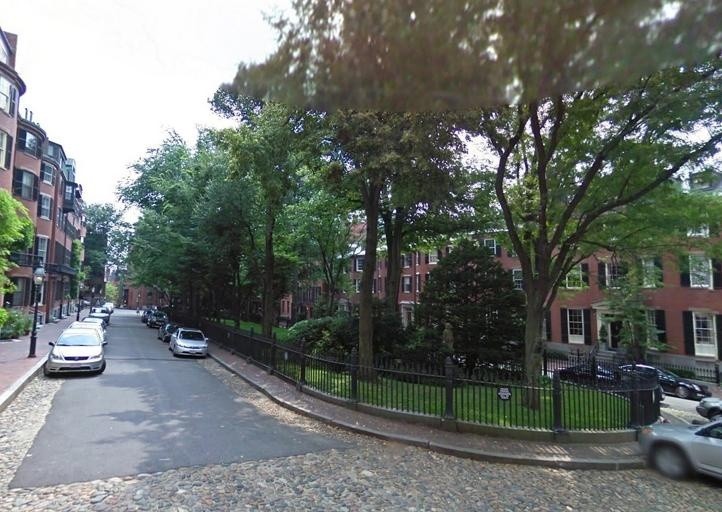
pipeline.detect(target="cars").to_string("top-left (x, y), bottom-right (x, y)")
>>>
top-left (551, 360), bottom-right (665, 402)
top-left (167, 326), bottom-right (209, 359)
top-left (635, 417), bottom-right (722, 485)
top-left (64, 300), bottom-right (115, 345)
top-left (140, 302), bottom-right (184, 343)
top-left (696, 395), bottom-right (722, 422)
top-left (43, 325), bottom-right (108, 377)
top-left (617, 361), bottom-right (714, 402)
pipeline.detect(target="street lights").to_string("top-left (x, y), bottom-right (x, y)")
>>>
top-left (76, 276), bottom-right (86, 321)
top-left (98, 287), bottom-right (103, 303)
top-left (89, 285), bottom-right (97, 313)
top-left (26, 259), bottom-right (48, 358)
